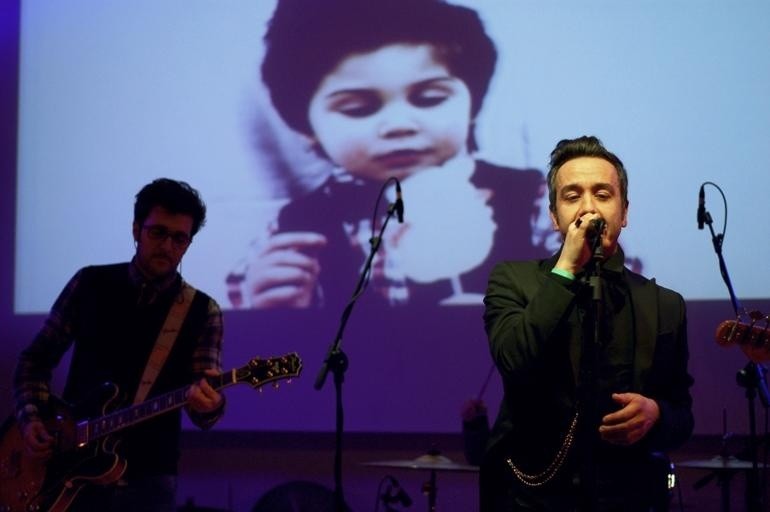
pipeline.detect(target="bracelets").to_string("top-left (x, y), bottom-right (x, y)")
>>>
top-left (551, 266), bottom-right (575, 280)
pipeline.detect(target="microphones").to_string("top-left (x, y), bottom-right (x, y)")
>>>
top-left (385, 470), bottom-right (413, 506)
top-left (392, 178), bottom-right (405, 223)
top-left (694, 188), bottom-right (708, 232)
top-left (584, 217), bottom-right (609, 249)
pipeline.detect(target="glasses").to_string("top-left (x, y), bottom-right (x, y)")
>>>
top-left (141, 224), bottom-right (192, 247)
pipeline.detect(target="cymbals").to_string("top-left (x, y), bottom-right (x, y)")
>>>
top-left (368, 456), bottom-right (481, 472)
top-left (675, 460), bottom-right (769, 468)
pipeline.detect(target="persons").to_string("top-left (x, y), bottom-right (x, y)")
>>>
top-left (252, 475), bottom-right (349, 512)
top-left (12, 176), bottom-right (227, 510)
top-left (481, 136), bottom-right (694, 512)
top-left (224, 0), bottom-right (550, 313)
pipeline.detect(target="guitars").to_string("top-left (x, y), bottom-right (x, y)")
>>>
top-left (716, 308), bottom-right (770, 362)
top-left (0, 353), bottom-right (304, 510)
